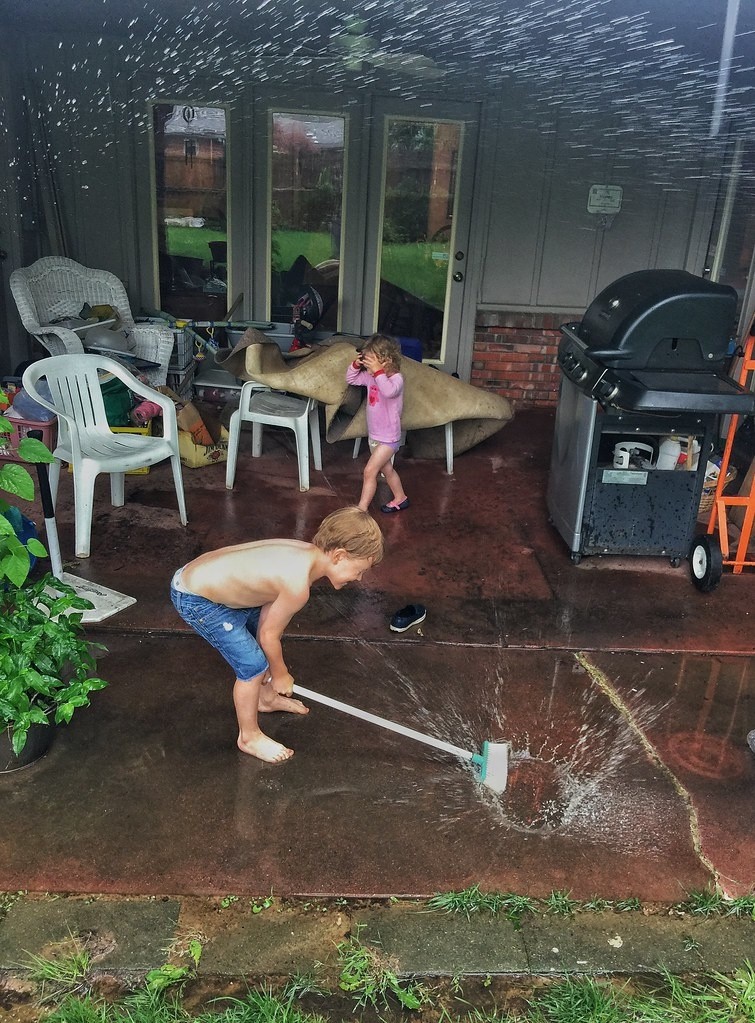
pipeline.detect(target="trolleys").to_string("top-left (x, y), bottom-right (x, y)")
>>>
top-left (689, 317), bottom-right (755, 593)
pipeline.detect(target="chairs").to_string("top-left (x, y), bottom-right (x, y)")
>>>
top-left (224, 380), bottom-right (322, 492)
top-left (22, 353), bottom-right (189, 558)
top-left (11, 256), bottom-right (175, 390)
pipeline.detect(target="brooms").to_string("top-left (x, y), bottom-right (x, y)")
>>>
top-left (267, 675), bottom-right (509, 796)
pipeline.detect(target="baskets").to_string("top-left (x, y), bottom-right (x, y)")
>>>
top-left (699, 463), bottom-right (737, 513)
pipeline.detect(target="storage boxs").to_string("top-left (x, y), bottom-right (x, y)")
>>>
top-left (165, 360), bottom-right (195, 409)
top-left (133, 315), bottom-right (194, 370)
top-left (0, 376), bottom-right (58, 463)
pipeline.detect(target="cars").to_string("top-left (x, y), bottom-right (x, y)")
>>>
top-left (161, 204), bottom-right (337, 292)
top-left (380, 225), bottom-right (453, 313)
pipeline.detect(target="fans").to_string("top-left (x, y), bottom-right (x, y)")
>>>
top-left (246, 13), bottom-right (448, 81)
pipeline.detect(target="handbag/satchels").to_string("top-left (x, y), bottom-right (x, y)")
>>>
top-left (167, 387), bottom-right (214, 445)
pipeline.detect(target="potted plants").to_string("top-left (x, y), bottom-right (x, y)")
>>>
top-left (1, 572), bottom-right (112, 776)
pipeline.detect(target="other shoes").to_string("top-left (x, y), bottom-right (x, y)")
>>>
top-left (390, 604), bottom-right (426, 633)
top-left (381, 496), bottom-right (409, 513)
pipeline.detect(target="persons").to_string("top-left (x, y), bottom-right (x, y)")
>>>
top-left (345, 333), bottom-right (409, 511)
top-left (169, 507), bottom-right (384, 764)
